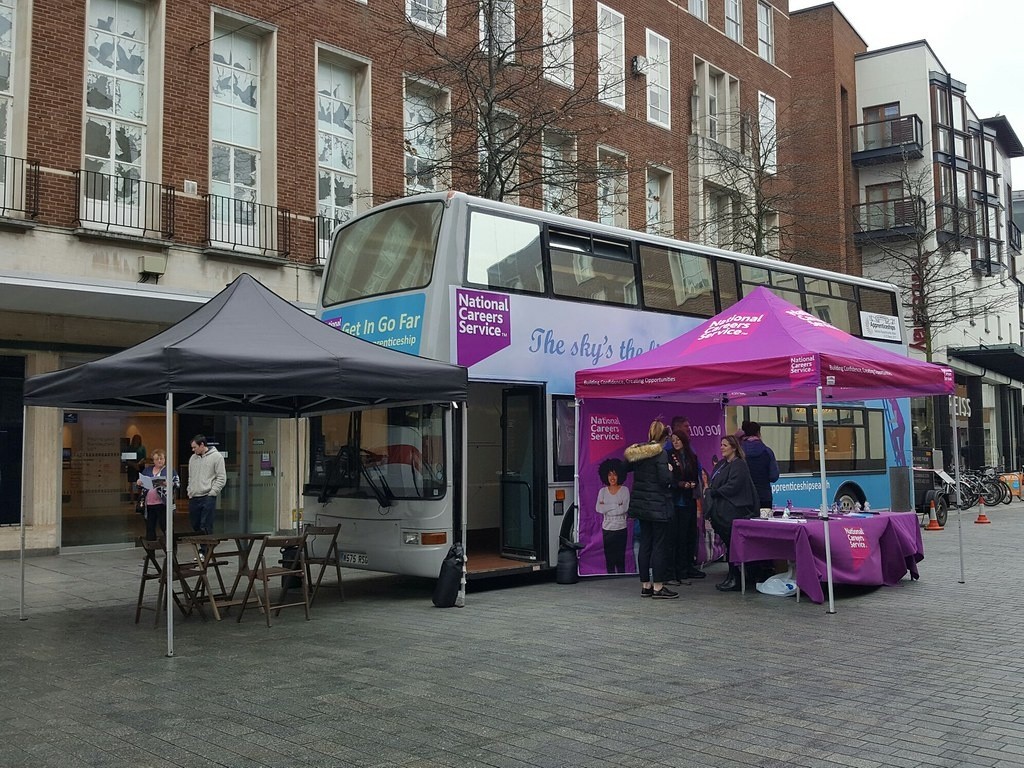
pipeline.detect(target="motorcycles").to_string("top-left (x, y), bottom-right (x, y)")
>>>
top-left (911, 446), bottom-right (947, 526)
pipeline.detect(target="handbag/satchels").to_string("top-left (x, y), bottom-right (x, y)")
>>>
top-left (135, 494), bottom-right (145, 513)
top-left (756, 564), bottom-right (797, 596)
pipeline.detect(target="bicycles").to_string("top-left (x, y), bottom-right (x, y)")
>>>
top-left (945, 464), bottom-right (1012, 510)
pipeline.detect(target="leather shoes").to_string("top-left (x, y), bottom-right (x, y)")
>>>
top-left (641, 585), bottom-right (654, 597)
top-left (652, 587), bottom-right (679, 599)
top-left (688, 570), bottom-right (706, 579)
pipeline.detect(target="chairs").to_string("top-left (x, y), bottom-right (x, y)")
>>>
top-left (134, 522), bottom-right (344, 628)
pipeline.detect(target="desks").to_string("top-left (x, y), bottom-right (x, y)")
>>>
top-left (728, 507), bottom-right (925, 603)
top-left (181, 532), bottom-right (272, 621)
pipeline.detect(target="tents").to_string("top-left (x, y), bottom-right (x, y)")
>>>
top-left (19, 273), bottom-right (469, 657)
top-left (573, 285), bottom-right (966, 614)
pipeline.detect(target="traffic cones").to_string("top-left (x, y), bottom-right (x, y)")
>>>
top-left (924, 500), bottom-right (944, 529)
top-left (974, 497), bottom-right (990, 523)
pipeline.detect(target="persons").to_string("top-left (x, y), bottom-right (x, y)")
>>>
top-left (622, 415), bottom-right (780, 599)
top-left (186, 434), bottom-right (227, 560)
top-left (595, 458), bottom-right (631, 573)
top-left (135, 449), bottom-right (181, 558)
top-left (125, 435), bottom-right (146, 503)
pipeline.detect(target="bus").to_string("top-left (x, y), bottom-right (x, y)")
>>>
top-left (302, 191), bottom-right (917, 586)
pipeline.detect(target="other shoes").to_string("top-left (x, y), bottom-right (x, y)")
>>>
top-left (678, 580), bottom-right (691, 585)
top-left (194, 553), bottom-right (205, 562)
top-left (664, 580), bottom-right (680, 585)
top-left (715, 571), bottom-right (741, 591)
top-left (143, 555), bottom-right (147, 560)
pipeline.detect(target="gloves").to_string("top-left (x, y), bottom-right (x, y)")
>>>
top-left (710, 488), bottom-right (719, 499)
